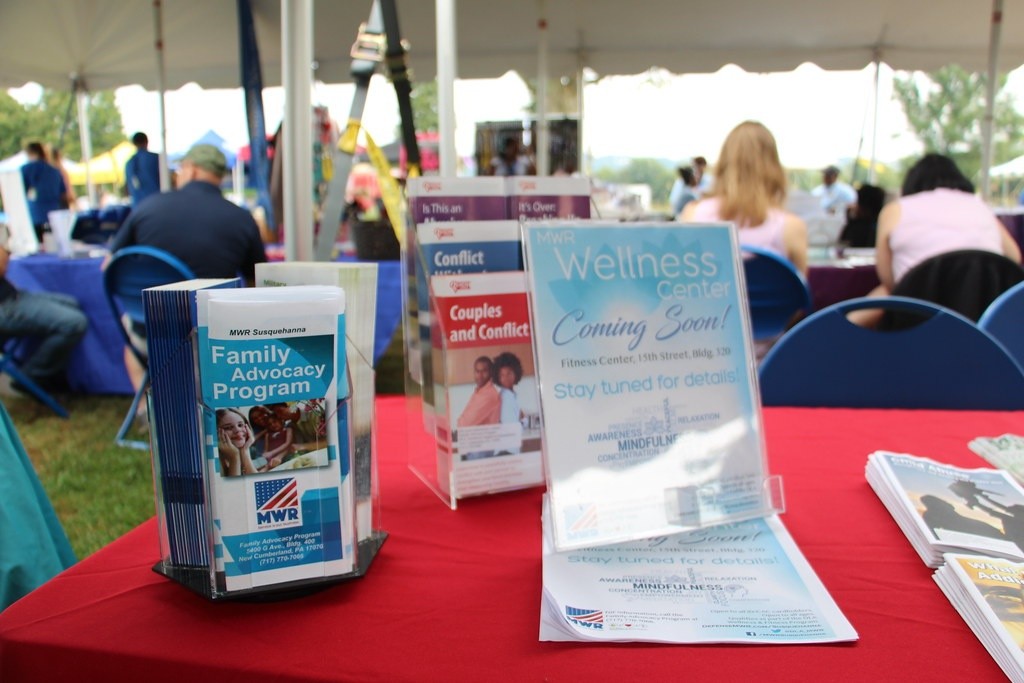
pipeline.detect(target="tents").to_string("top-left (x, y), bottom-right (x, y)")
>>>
top-left (0, 0), bottom-right (1024, 203)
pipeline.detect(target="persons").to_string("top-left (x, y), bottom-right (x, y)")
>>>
top-left (456, 352), bottom-right (524, 428)
top-left (0, 221), bottom-right (89, 422)
top-left (845, 154), bottom-right (1022, 329)
top-left (669, 121), bottom-right (885, 366)
top-left (22, 142), bottom-right (76, 252)
top-left (125, 132), bottom-right (160, 211)
top-left (215, 403), bottom-right (326, 478)
top-left (100, 145), bottom-right (268, 436)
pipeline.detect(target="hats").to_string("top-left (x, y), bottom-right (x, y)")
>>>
top-left (172, 144), bottom-right (226, 177)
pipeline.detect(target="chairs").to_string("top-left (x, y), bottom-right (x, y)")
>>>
top-left (737, 244), bottom-right (1024, 410)
top-left (102, 245), bottom-right (196, 452)
top-left (0, 336), bottom-right (68, 418)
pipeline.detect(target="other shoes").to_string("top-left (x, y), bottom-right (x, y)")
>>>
top-left (10, 378), bottom-right (61, 402)
top-left (134, 412), bottom-right (148, 437)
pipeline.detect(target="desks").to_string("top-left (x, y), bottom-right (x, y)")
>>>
top-left (5, 245), bottom-right (245, 396)
top-left (331, 254), bottom-right (401, 366)
top-left (0, 397), bottom-right (1024, 682)
top-left (808, 247), bottom-right (880, 312)
